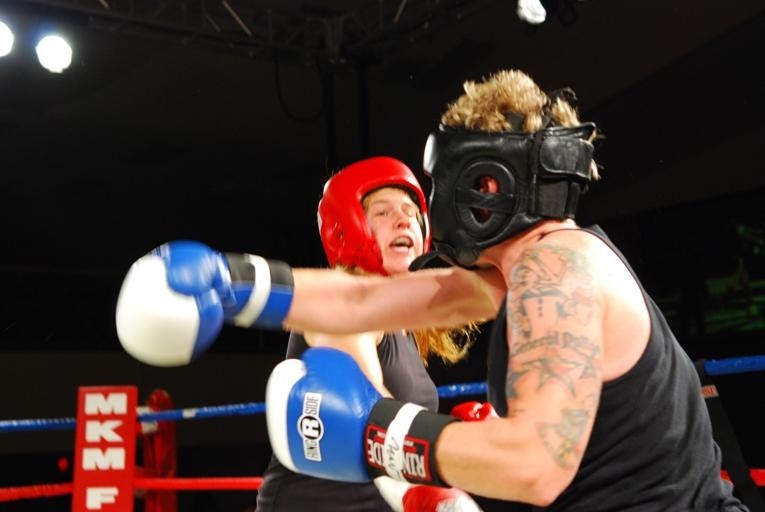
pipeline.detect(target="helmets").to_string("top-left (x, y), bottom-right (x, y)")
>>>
top-left (317, 157), bottom-right (432, 276)
top-left (423, 88), bottom-right (595, 270)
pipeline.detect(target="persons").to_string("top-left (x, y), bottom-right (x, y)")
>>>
top-left (115, 67), bottom-right (750, 512)
top-left (254, 155), bottom-right (501, 512)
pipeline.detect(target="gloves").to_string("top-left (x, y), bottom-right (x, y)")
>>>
top-left (374, 477), bottom-right (482, 512)
top-left (116, 242), bottom-right (292, 368)
top-left (451, 402), bottom-right (499, 422)
top-left (266, 348), bottom-right (460, 483)
